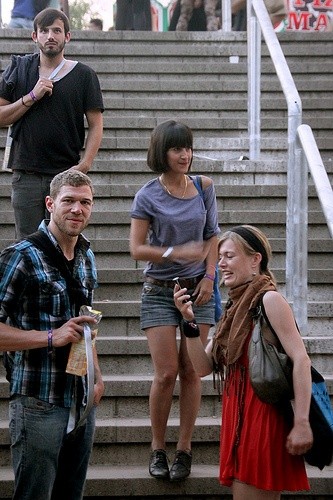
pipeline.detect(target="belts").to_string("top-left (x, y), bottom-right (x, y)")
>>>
top-left (145, 274), bottom-right (205, 289)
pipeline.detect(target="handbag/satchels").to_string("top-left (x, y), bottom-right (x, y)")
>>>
top-left (303, 366), bottom-right (333, 470)
top-left (248, 290), bottom-right (294, 404)
top-left (2, 352), bottom-right (13, 382)
top-left (215, 262), bottom-right (222, 322)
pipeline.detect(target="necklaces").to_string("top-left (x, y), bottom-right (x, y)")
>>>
top-left (159, 174), bottom-right (188, 200)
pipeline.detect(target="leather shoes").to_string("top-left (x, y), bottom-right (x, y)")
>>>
top-left (169, 450), bottom-right (192, 482)
top-left (149, 446), bottom-right (169, 478)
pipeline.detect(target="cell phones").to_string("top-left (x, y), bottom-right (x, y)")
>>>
top-left (172, 277), bottom-right (189, 303)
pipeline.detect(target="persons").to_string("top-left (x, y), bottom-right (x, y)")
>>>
top-left (1, 171), bottom-right (106, 500)
top-left (128, 121), bottom-right (221, 483)
top-left (3, 1), bottom-right (289, 30)
top-left (7, 0), bottom-right (50, 32)
top-left (170, 0), bottom-right (223, 32)
top-left (0, 8), bottom-right (106, 240)
top-left (85, 18), bottom-right (106, 31)
top-left (172, 224), bottom-right (316, 500)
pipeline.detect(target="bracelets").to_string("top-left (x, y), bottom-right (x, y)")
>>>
top-left (203, 273), bottom-right (215, 281)
top-left (21, 90), bottom-right (38, 107)
top-left (161, 247), bottom-right (174, 260)
top-left (47, 328), bottom-right (54, 350)
top-left (206, 264), bottom-right (217, 270)
